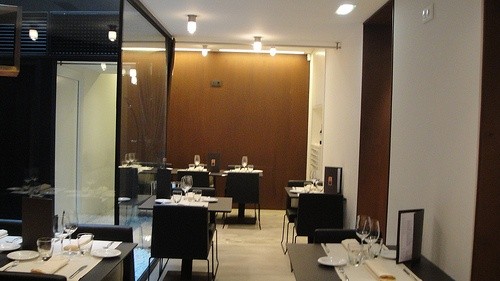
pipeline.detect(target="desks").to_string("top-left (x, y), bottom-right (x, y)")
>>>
top-left (138, 195), bottom-right (233, 281)
top-left (284, 186), bottom-right (348, 203)
top-left (286, 243), bottom-right (456, 281)
top-left (0, 237), bottom-right (139, 281)
top-left (170, 168), bottom-right (263, 225)
top-left (138, 167), bottom-right (157, 215)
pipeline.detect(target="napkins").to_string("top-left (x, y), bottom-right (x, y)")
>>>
top-left (183, 192), bottom-right (195, 201)
top-left (31, 255), bottom-right (71, 274)
top-left (341, 238), bottom-right (361, 254)
top-left (364, 257), bottom-right (397, 281)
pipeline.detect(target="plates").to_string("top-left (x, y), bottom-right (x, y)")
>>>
top-left (155, 199), bottom-right (172, 202)
top-left (91, 249), bottom-right (121, 257)
top-left (318, 257), bottom-right (347, 265)
top-left (202, 198), bottom-right (218, 201)
top-left (7, 250), bottom-right (39, 260)
top-left (381, 250), bottom-right (396, 259)
top-left (0, 242), bottom-right (20, 250)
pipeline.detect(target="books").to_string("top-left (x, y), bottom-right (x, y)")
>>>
top-left (208, 152), bottom-right (221, 173)
top-left (22, 196), bottom-right (54, 252)
top-left (325, 167), bottom-right (342, 196)
top-left (157, 168), bottom-right (171, 200)
top-left (396, 209), bottom-right (424, 264)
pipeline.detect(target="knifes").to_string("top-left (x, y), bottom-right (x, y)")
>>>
top-left (69, 265), bottom-right (88, 279)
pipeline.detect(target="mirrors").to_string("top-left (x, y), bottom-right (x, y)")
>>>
top-left (119, 0), bottom-right (170, 249)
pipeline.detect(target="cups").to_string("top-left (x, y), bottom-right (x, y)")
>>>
top-left (193, 190), bottom-right (202, 202)
top-left (37, 238), bottom-right (54, 262)
top-left (369, 237), bottom-right (383, 258)
top-left (348, 243), bottom-right (363, 267)
top-left (189, 164), bottom-right (204, 170)
top-left (77, 233), bottom-right (93, 257)
top-left (235, 165), bottom-right (253, 172)
top-left (122, 161), bottom-right (139, 167)
top-left (173, 191), bottom-right (182, 203)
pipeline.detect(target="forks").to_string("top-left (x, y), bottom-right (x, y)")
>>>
top-left (3, 261), bottom-right (19, 271)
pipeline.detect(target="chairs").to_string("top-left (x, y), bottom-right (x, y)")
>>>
top-left (63, 224), bottom-right (135, 281)
top-left (177, 171), bottom-right (210, 188)
top-left (222, 172), bottom-right (261, 230)
top-left (148, 206), bottom-right (215, 281)
top-left (281, 179), bottom-right (313, 255)
top-left (172, 188), bottom-right (220, 277)
top-left (308, 228), bottom-right (383, 245)
top-left (118, 166), bottom-right (152, 225)
top-left (290, 192), bottom-right (345, 274)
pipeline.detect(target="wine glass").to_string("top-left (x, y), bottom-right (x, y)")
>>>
top-left (356, 215), bottom-right (371, 245)
top-left (242, 157), bottom-right (247, 171)
top-left (53, 214), bottom-right (71, 258)
top-left (125, 153), bottom-right (134, 167)
top-left (363, 219), bottom-right (380, 260)
top-left (62, 210), bottom-right (79, 255)
top-left (181, 176), bottom-right (193, 202)
top-left (194, 155), bottom-right (200, 168)
